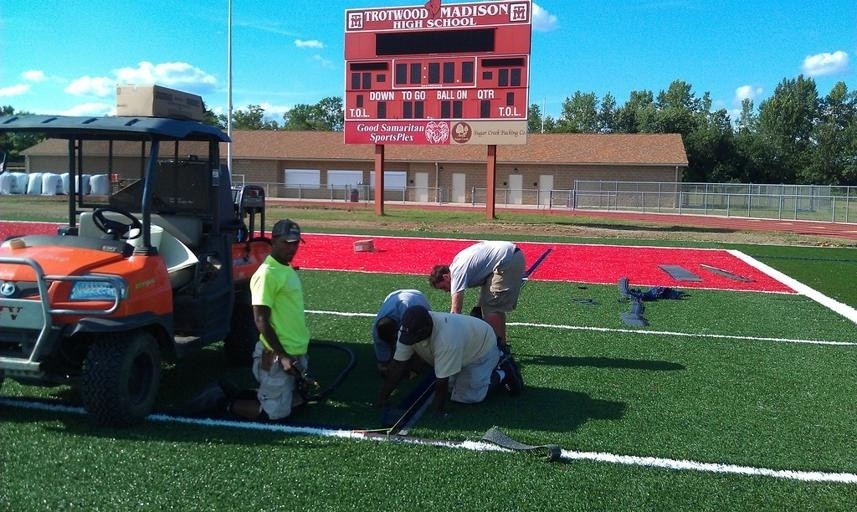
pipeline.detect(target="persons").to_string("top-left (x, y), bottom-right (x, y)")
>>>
top-left (188, 218), bottom-right (312, 424)
top-left (372, 288), bottom-right (433, 385)
top-left (366, 304), bottom-right (525, 419)
top-left (429, 240), bottom-right (524, 365)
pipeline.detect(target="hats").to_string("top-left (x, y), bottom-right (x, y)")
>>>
top-left (399, 305), bottom-right (433, 345)
top-left (272, 219), bottom-right (305, 244)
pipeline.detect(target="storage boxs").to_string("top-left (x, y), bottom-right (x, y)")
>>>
top-left (353, 240), bottom-right (374, 251)
top-left (115, 84), bottom-right (206, 122)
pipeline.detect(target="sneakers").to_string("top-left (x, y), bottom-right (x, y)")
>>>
top-left (502, 357), bottom-right (523, 396)
top-left (191, 381), bottom-right (240, 408)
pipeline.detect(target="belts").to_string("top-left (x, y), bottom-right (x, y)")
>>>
top-left (514, 247), bottom-right (520, 254)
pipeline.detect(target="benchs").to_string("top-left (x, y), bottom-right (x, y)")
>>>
top-left (79, 212), bottom-right (203, 252)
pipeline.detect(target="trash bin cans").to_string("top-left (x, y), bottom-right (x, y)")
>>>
top-left (350, 189), bottom-right (358, 202)
